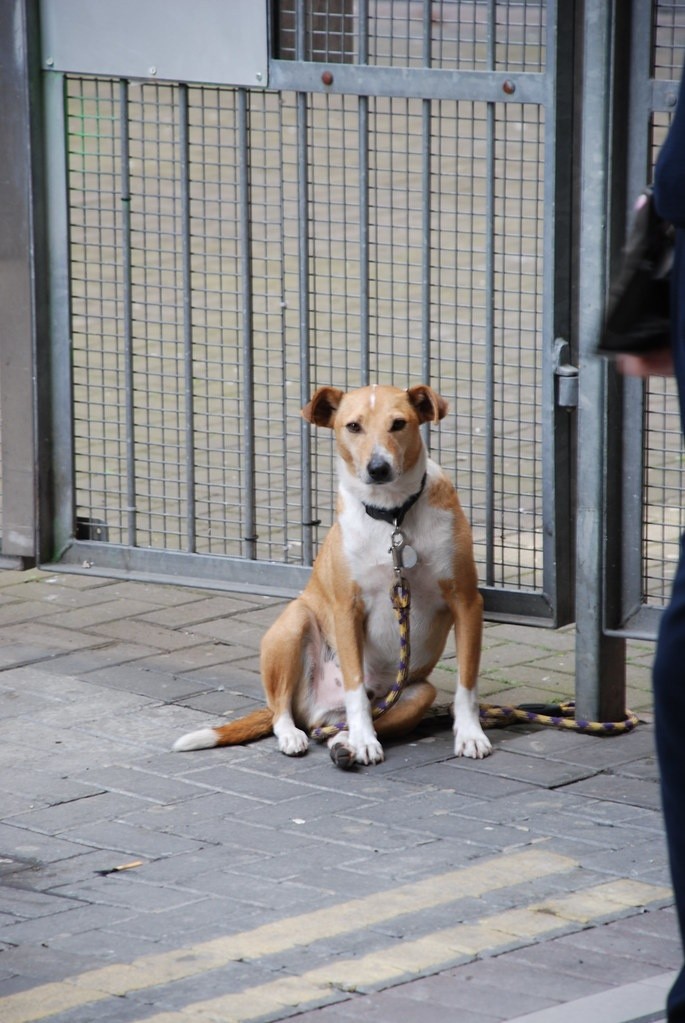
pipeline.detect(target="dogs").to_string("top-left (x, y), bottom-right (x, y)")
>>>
top-left (172, 384), bottom-right (496, 774)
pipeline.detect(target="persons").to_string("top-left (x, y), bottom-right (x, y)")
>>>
top-left (615, 61), bottom-right (685, 1023)
